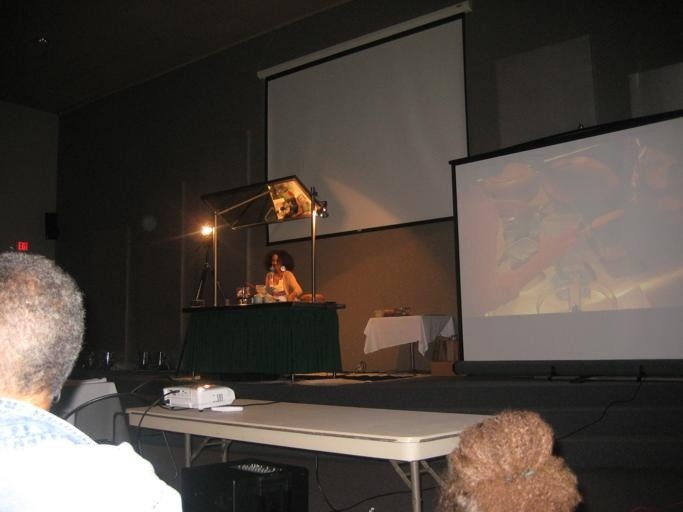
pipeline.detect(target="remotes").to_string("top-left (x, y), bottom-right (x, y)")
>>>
top-left (211, 406), bottom-right (243, 412)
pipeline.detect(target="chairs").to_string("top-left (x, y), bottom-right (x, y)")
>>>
top-left (61, 378), bottom-right (182, 481)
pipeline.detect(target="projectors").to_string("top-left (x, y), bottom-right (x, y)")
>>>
top-left (164, 384), bottom-right (236, 411)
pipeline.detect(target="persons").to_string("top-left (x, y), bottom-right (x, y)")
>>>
top-left (244, 250), bottom-right (303, 302)
top-left (0, 248), bottom-right (185, 512)
top-left (436, 405), bottom-right (586, 512)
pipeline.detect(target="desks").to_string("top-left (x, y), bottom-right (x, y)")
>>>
top-left (181, 300), bottom-right (346, 382)
top-left (125, 399), bottom-right (494, 512)
top-left (363, 315), bottom-right (454, 371)
top-left (52, 380), bottom-right (116, 431)
